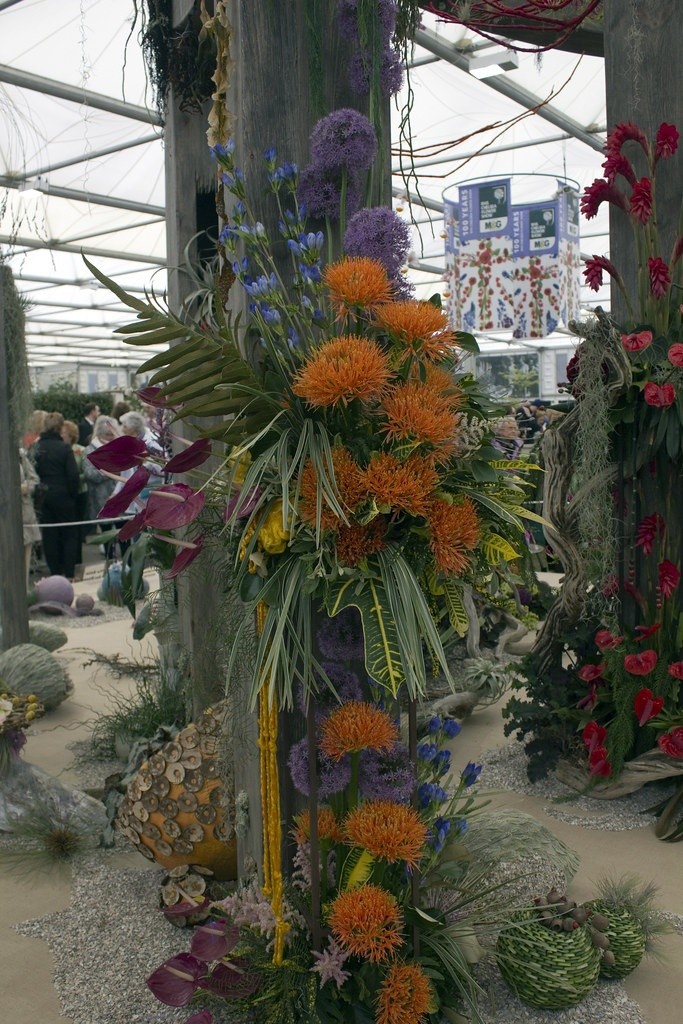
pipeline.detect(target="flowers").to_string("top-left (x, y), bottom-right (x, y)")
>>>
top-left (80, 0), bottom-right (534, 1024)
top-left (566, 119), bottom-right (683, 781)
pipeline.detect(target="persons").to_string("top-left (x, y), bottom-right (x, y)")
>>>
top-left (16, 400), bottom-right (170, 593)
top-left (487, 399), bottom-right (559, 484)
